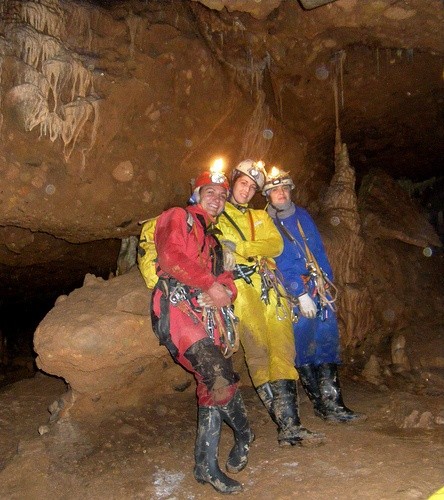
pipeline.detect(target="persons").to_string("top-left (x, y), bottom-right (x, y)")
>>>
top-left (215, 157), bottom-right (331, 449)
top-left (149, 170), bottom-right (256, 494)
top-left (262, 167), bottom-right (370, 425)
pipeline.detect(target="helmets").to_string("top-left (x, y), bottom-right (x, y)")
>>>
top-left (235, 159), bottom-right (266, 193)
top-left (262, 166), bottom-right (295, 196)
top-left (192, 171), bottom-right (229, 198)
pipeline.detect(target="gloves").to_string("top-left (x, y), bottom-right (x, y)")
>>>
top-left (195, 288), bottom-right (232, 309)
top-left (297, 293), bottom-right (318, 319)
top-left (223, 252), bottom-right (236, 271)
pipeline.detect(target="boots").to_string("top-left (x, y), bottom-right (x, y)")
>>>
top-left (254, 378), bottom-right (327, 448)
top-left (313, 363), bottom-right (368, 424)
top-left (294, 362), bottom-right (334, 419)
top-left (192, 405), bottom-right (243, 494)
top-left (217, 389), bottom-right (255, 473)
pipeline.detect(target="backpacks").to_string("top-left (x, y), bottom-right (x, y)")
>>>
top-left (137, 212), bottom-right (220, 289)
